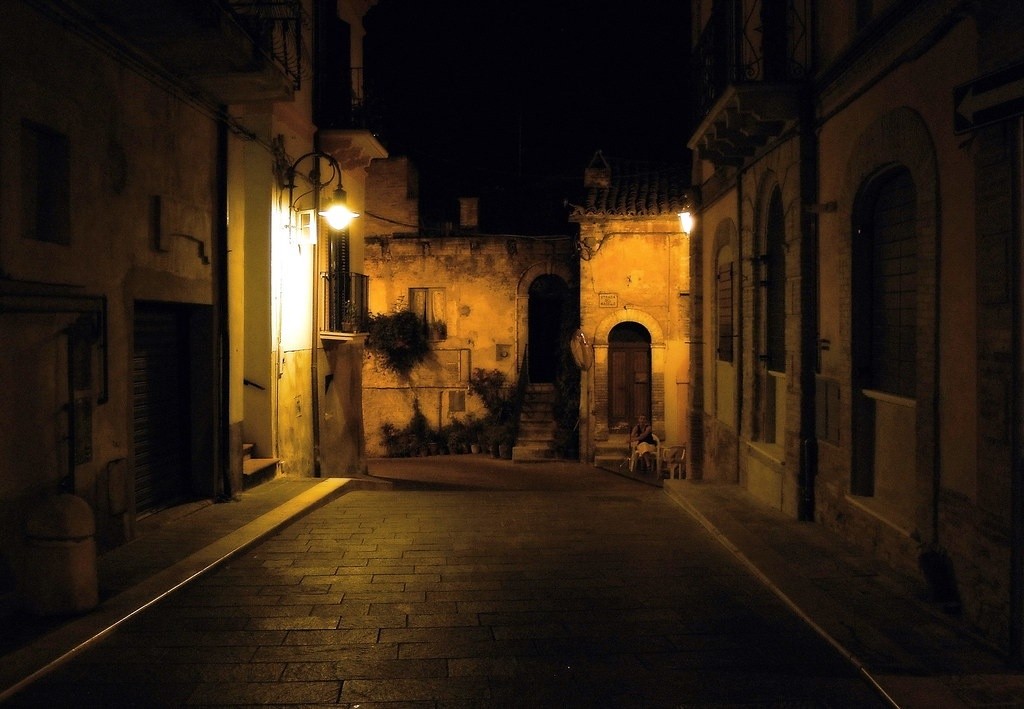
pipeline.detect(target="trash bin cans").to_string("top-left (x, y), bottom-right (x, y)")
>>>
top-left (12, 494), bottom-right (99, 617)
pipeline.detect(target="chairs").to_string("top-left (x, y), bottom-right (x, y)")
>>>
top-left (629, 432), bottom-right (661, 478)
top-left (663, 445), bottom-right (687, 479)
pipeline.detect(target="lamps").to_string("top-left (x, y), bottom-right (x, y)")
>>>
top-left (277, 138), bottom-right (362, 231)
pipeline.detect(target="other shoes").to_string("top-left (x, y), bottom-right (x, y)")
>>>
top-left (647, 463), bottom-right (651, 470)
top-left (640, 465), bottom-right (645, 471)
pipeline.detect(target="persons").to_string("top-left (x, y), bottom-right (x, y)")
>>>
top-left (630, 413), bottom-right (657, 472)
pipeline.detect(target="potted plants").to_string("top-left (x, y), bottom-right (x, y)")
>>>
top-left (341, 300), bottom-right (513, 458)
